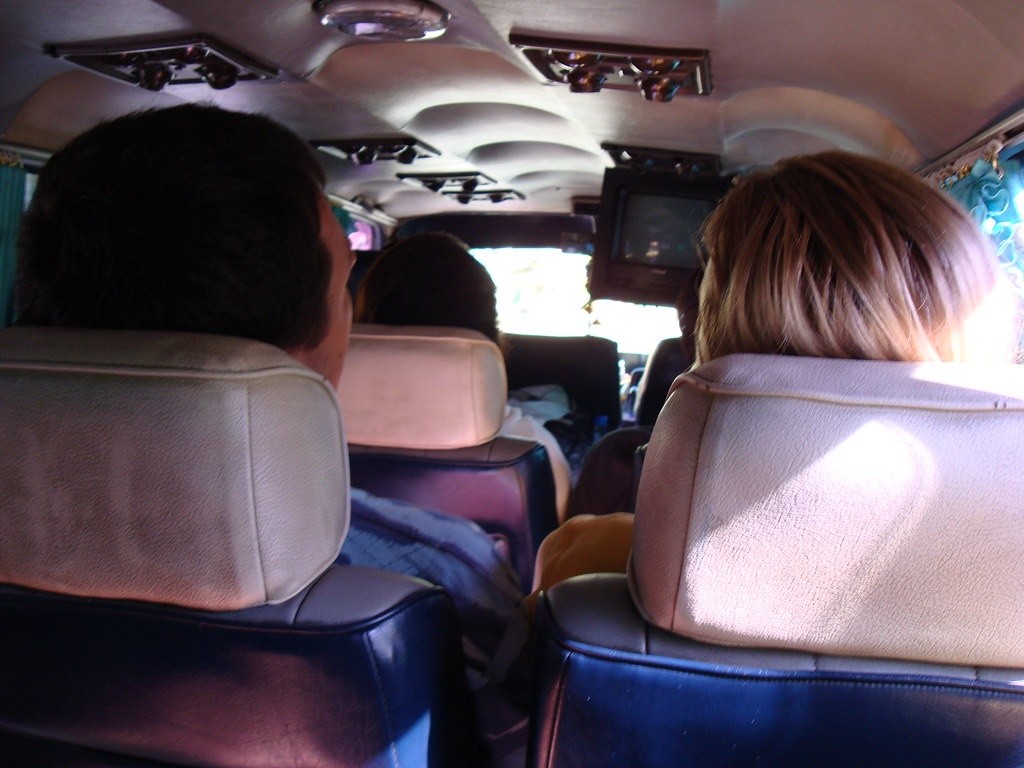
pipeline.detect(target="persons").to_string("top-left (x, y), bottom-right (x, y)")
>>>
top-left (531, 147), bottom-right (1024, 592)
top-left (10, 100), bottom-right (540, 768)
top-left (351, 228), bottom-right (570, 527)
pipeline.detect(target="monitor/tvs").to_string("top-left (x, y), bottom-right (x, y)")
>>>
top-left (610, 187), bottom-right (720, 290)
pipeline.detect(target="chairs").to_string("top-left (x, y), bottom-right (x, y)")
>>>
top-left (534, 337), bottom-right (1024, 768)
top-left (0, 326), bottom-right (495, 768)
top-left (499, 333), bottom-right (619, 443)
top-left (334, 325), bottom-right (561, 596)
